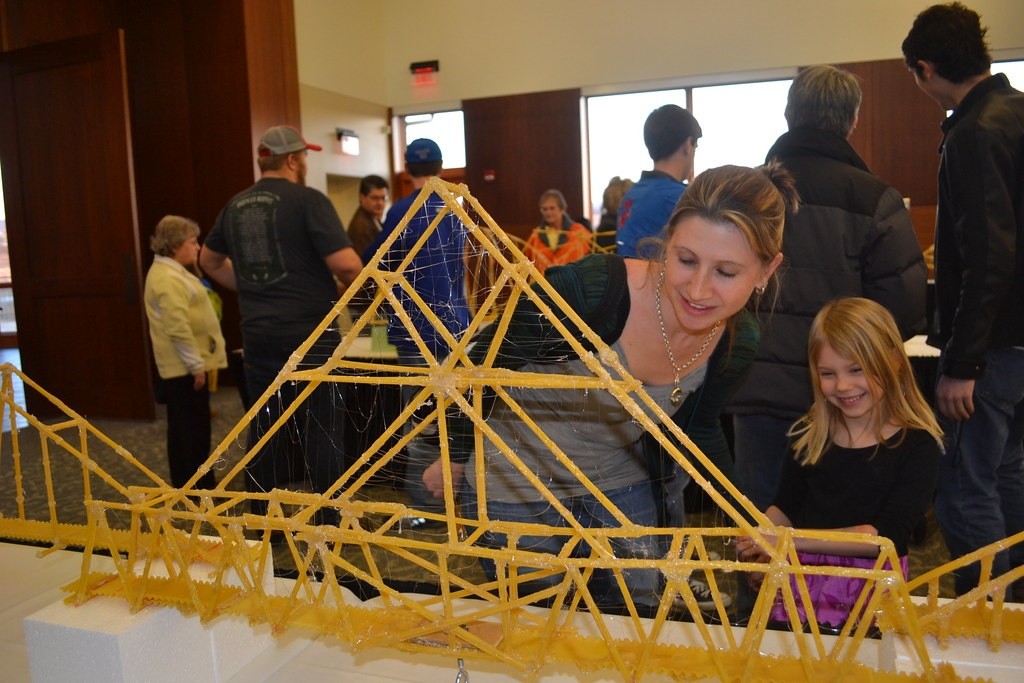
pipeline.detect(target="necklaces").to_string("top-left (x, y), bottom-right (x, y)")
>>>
top-left (656, 268), bottom-right (722, 406)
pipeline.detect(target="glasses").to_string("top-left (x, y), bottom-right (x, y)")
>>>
top-left (367, 195), bottom-right (389, 201)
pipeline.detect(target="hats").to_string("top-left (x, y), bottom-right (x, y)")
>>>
top-left (258, 125), bottom-right (322, 157)
top-left (404, 138), bottom-right (442, 162)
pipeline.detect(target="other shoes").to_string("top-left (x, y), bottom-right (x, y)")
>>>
top-left (383, 515), bottom-right (449, 536)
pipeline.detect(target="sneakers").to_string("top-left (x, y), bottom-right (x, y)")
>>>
top-left (679, 548), bottom-right (722, 563)
top-left (671, 578), bottom-right (733, 611)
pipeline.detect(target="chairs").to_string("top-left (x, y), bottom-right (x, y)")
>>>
top-left (230, 353), bottom-right (247, 415)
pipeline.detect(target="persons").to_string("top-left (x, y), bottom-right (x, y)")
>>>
top-left (616, 104), bottom-right (733, 613)
top-left (359, 138), bottom-right (476, 529)
top-left (597, 173), bottom-right (640, 255)
top-left (519, 189), bottom-right (592, 287)
top-left (200, 126), bottom-right (364, 551)
top-left (900, 0), bottom-right (1024, 604)
top-left (347, 175), bottom-right (390, 308)
top-left (718, 64), bottom-right (938, 627)
top-left (142, 215), bottom-right (230, 500)
top-left (422, 154), bottom-right (801, 619)
top-left (733, 295), bottom-right (946, 636)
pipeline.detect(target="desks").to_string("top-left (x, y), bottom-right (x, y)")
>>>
top-left (0, 541), bottom-right (881, 683)
top-left (340, 337), bottom-right (409, 489)
